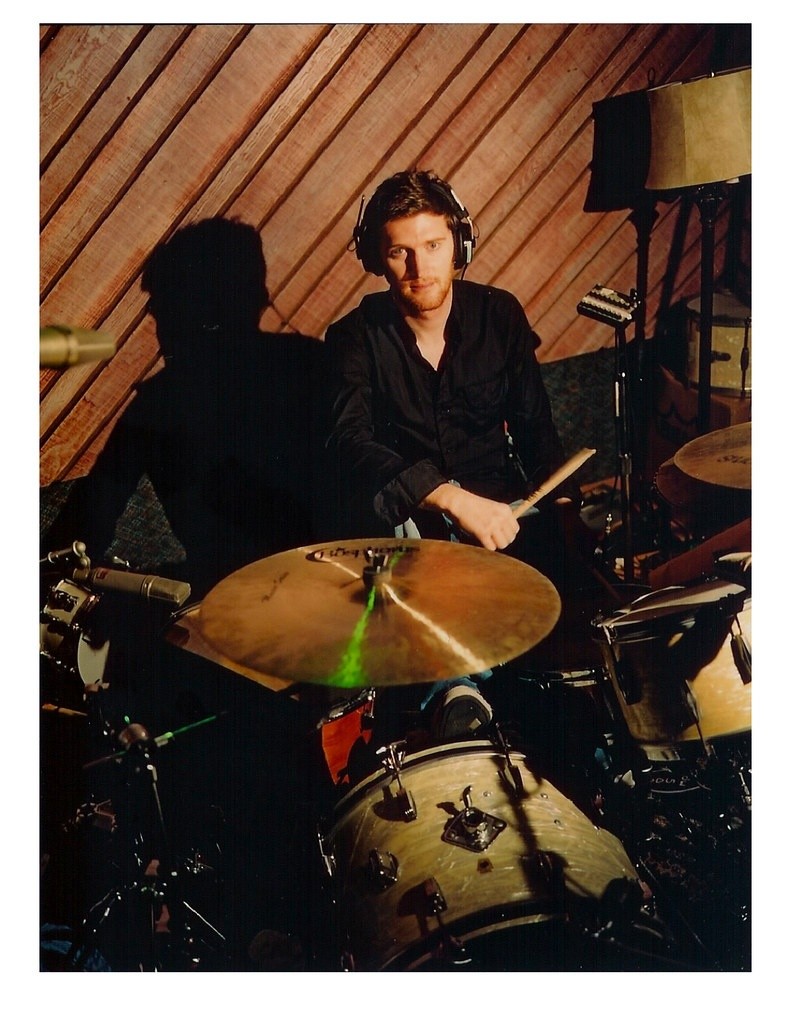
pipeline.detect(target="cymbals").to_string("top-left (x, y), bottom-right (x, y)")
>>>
top-left (673, 421), bottom-right (751, 489)
top-left (654, 456), bottom-right (752, 520)
top-left (194, 536), bottom-right (562, 691)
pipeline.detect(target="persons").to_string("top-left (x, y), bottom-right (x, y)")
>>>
top-left (312, 172), bottom-right (582, 573)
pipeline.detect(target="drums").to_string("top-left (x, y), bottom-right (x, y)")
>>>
top-left (588, 573), bottom-right (752, 744)
top-left (161, 599), bottom-right (304, 698)
top-left (307, 738), bottom-right (701, 972)
top-left (518, 580), bottom-right (707, 793)
top-left (686, 286), bottom-right (752, 399)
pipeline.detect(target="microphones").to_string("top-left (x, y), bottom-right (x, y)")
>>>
top-left (72, 568), bottom-right (192, 607)
top-left (40, 330), bottom-right (117, 370)
top-left (353, 195), bottom-right (365, 247)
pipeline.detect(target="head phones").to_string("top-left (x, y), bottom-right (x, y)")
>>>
top-left (358, 182), bottom-right (476, 277)
